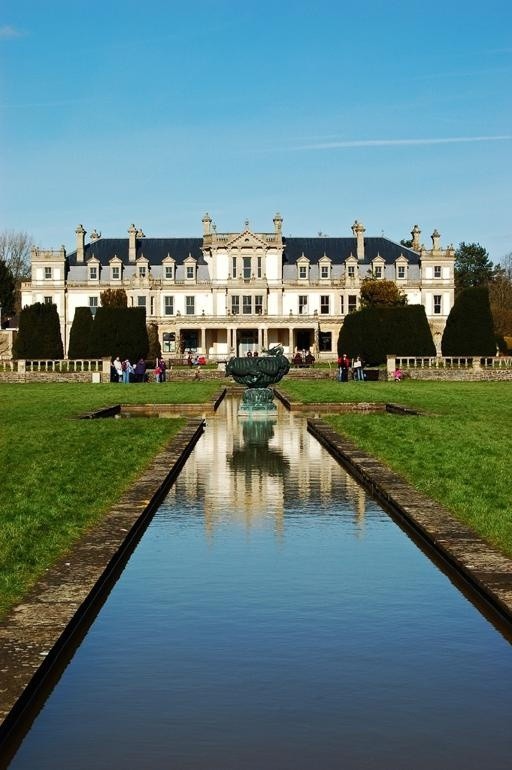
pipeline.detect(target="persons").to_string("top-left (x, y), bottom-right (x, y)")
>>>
top-left (254, 350), bottom-right (258, 356)
top-left (305, 351), bottom-right (315, 368)
top-left (338, 353), bottom-right (350, 382)
top-left (393, 367), bottom-right (403, 379)
top-left (111, 356), bottom-right (168, 385)
top-left (186, 351), bottom-right (199, 366)
top-left (294, 353), bottom-right (302, 368)
top-left (247, 349), bottom-right (252, 357)
top-left (353, 353), bottom-right (365, 381)
